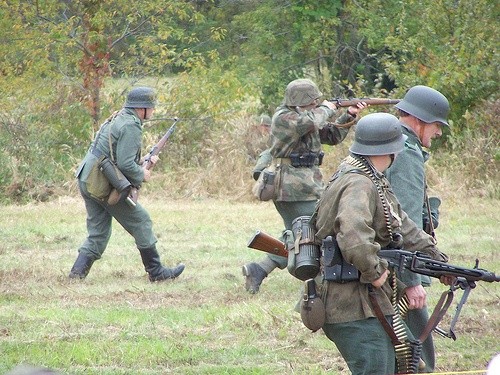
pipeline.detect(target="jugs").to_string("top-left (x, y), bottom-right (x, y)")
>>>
top-left (301, 279), bottom-right (325, 331)
top-left (254, 171), bottom-right (276, 201)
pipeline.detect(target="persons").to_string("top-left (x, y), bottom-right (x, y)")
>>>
top-left (293, 113), bottom-right (458, 375)
top-left (66, 85), bottom-right (185, 281)
top-left (381, 85), bottom-right (450, 372)
top-left (242, 78), bottom-right (371, 294)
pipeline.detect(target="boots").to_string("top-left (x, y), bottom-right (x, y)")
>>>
top-left (138, 244), bottom-right (185, 281)
top-left (69, 254), bottom-right (95, 279)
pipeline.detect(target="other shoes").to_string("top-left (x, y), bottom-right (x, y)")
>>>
top-left (242, 262), bottom-right (268, 293)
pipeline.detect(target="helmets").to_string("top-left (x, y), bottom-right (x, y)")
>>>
top-left (349, 112), bottom-right (407, 155)
top-left (123, 87), bottom-right (155, 108)
top-left (285, 78), bottom-right (323, 106)
top-left (393, 85), bottom-right (450, 129)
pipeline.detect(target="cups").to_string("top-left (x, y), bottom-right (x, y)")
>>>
top-left (97, 158), bottom-right (131, 193)
top-left (281, 215), bottom-right (321, 282)
top-left (252, 150), bottom-right (272, 180)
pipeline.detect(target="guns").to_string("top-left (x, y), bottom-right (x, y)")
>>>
top-left (296, 97), bottom-right (402, 116)
top-left (125, 116), bottom-right (179, 209)
top-left (246, 228), bottom-right (455, 340)
top-left (322, 236), bottom-right (500, 287)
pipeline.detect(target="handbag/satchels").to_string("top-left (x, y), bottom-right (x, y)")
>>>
top-left (252, 168), bottom-right (281, 199)
top-left (87, 159), bottom-right (112, 202)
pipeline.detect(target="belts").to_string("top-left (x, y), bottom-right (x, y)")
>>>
top-left (90, 145), bottom-right (108, 160)
